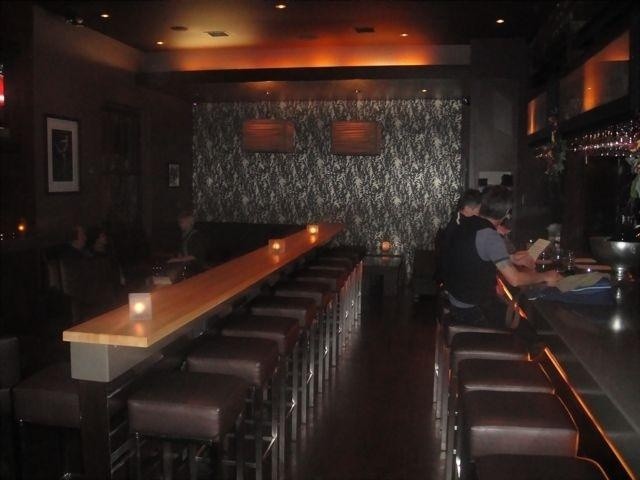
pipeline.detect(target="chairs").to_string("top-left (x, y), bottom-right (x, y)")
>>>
top-left (407, 249), bottom-right (442, 304)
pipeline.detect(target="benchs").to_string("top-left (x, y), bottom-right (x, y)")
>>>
top-left (0, 222), bottom-right (307, 337)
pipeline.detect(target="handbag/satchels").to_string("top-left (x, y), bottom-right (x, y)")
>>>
top-left (553, 272), bottom-right (612, 293)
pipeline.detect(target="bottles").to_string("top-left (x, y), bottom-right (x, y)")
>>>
top-left (605, 230), bottom-right (640, 242)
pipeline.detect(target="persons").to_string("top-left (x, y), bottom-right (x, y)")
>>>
top-left (435, 184), bottom-right (565, 339)
top-left (71, 224), bottom-right (108, 254)
top-left (166, 210), bottom-right (206, 267)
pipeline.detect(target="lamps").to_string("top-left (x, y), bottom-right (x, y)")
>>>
top-left (306, 224), bottom-right (319, 234)
top-left (242, 119), bottom-right (294, 153)
top-left (268, 238), bottom-right (287, 254)
top-left (330, 120), bottom-right (382, 157)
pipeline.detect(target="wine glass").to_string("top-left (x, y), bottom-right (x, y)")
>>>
top-left (565, 121), bottom-right (640, 158)
top-left (589, 235), bottom-right (640, 289)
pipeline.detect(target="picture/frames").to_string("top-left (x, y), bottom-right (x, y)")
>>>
top-left (43, 113), bottom-right (80, 195)
top-left (167, 162), bottom-right (181, 188)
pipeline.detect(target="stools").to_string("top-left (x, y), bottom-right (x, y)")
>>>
top-left (12, 361), bottom-right (127, 479)
top-left (433, 295), bottom-right (609, 480)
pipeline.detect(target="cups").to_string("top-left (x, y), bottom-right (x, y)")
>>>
top-left (553, 250), bottom-right (576, 277)
